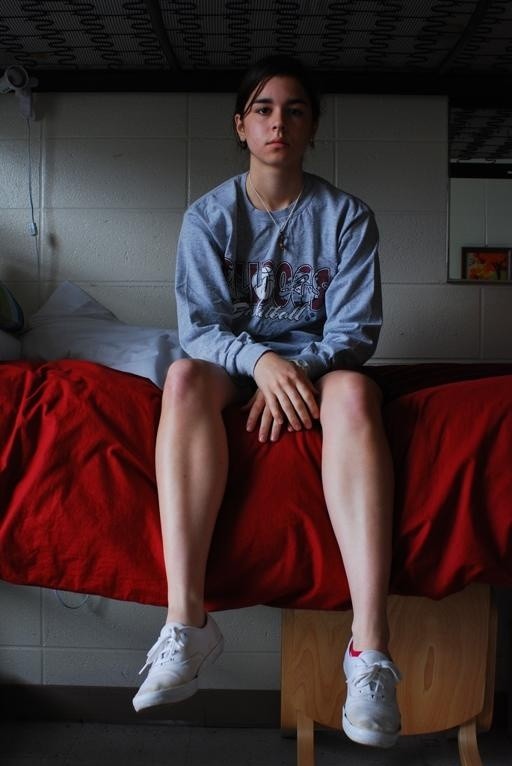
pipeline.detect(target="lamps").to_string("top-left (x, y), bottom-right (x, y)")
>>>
top-left (0, 65), bottom-right (34, 118)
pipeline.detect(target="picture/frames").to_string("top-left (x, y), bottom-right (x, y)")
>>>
top-left (460, 246), bottom-right (509, 281)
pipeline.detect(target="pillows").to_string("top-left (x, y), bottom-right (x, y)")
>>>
top-left (1, 285), bottom-right (40, 361)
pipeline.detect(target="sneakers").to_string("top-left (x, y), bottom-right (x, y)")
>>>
top-left (130, 612), bottom-right (227, 713)
top-left (339, 632), bottom-right (402, 749)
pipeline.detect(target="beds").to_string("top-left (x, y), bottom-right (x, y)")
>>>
top-left (0, 359), bottom-right (512, 732)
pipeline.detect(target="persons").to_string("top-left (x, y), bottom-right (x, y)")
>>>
top-left (129, 52), bottom-right (410, 751)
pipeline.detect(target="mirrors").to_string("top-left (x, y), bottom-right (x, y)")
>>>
top-left (446, 89), bottom-right (511, 285)
